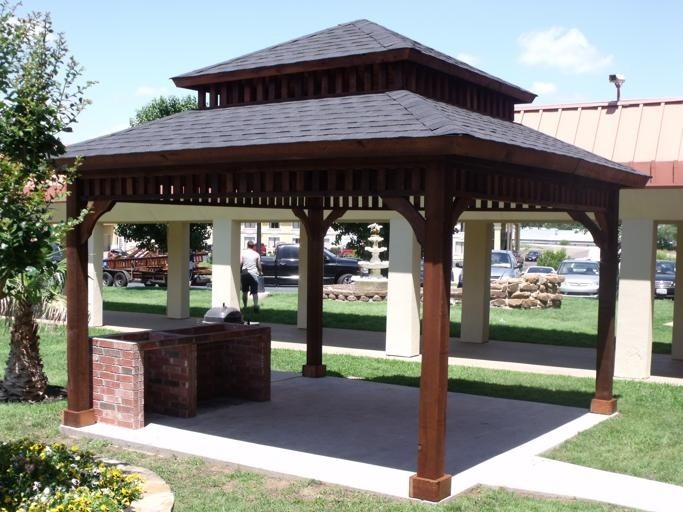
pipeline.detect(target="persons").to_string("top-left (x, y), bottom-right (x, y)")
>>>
top-left (240, 240), bottom-right (264, 314)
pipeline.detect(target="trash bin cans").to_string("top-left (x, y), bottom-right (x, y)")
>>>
top-left (202, 306), bottom-right (244, 323)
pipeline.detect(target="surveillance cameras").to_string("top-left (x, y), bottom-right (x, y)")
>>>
top-left (609, 73), bottom-right (625, 82)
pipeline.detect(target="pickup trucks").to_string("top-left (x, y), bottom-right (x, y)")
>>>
top-left (241, 243), bottom-right (369, 287)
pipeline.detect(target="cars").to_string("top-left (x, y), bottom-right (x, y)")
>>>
top-left (456, 250), bottom-right (600, 298)
top-left (655, 260), bottom-right (676, 298)
